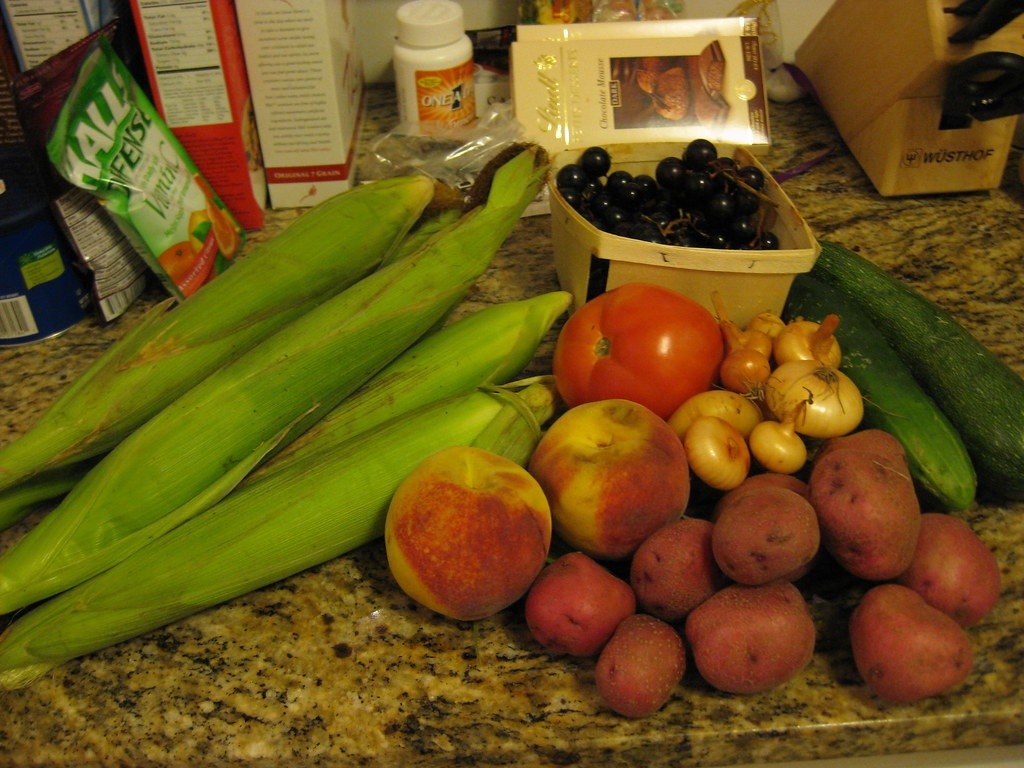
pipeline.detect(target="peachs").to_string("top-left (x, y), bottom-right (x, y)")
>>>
top-left (527, 398), bottom-right (690, 562)
top-left (384, 445), bottom-right (551, 621)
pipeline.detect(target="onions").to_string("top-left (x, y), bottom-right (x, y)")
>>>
top-left (665, 291), bottom-right (864, 489)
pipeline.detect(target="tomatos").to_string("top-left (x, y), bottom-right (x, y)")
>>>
top-left (551, 283), bottom-right (726, 422)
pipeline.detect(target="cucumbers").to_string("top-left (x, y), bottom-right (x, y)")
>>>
top-left (781, 240), bottom-right (1024, 513)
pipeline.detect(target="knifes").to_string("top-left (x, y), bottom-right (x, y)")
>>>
top-left (948, 0), bottom-right (1024, 42)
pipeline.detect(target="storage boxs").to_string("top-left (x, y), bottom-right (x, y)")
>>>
top-left (0, 0), bottom-right (367, 231)
top-left (509, 18), bottom-right (774, 164)
top-left (543, 141), bottom-right (819, 327)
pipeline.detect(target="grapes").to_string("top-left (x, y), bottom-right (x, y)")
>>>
top-left (553, 137), bottom-right (785, 302)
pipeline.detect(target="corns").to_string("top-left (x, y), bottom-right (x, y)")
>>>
top-left (0, 148), bottom-right (561, 689)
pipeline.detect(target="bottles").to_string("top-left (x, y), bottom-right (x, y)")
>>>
top-left (393, 0), bottom-right (476, 135)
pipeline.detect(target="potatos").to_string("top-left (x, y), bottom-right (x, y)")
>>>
top-left (526, 430), bottom-right (1000, 703)
